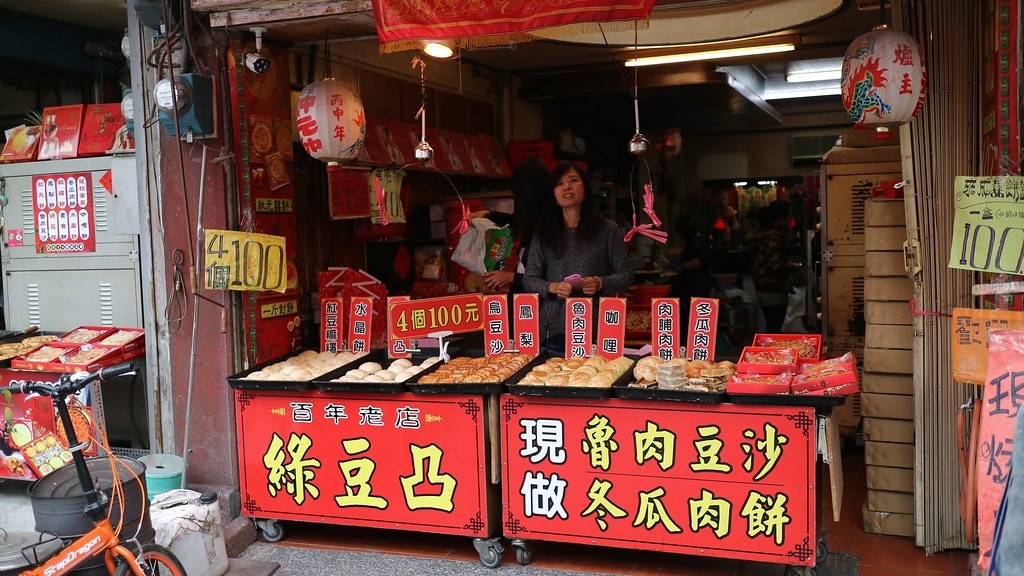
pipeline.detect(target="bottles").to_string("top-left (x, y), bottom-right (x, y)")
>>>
top-left (494, 259), bottom-right (506, 290)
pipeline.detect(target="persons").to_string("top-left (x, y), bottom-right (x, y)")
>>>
top-left (40, 123), bottom-right (59, 159)
top-left (522, 164), bottom-right (635, 346)
top-left (468, 159), bottom-right (549, 339)
top-left (751, 207), bottom-right (805, 333)
top-left (675, 213), bottom-right (714, 298)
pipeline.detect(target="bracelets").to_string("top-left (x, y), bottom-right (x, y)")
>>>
top-left (594, 275), bottom-right (602, 292)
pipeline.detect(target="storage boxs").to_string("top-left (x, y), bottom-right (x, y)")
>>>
top-left (861, 196), bottom-right (919, 539)
top-left (10, 326), bottom-right (147, 373)
top-left (358, 114), bottom-right (674, 344)
top-left (728, 332), bottom-right (858, 401)
top-left (0, 102), bottom-right (125, 166)
top-left (821, 126), bottom-right (903, 164)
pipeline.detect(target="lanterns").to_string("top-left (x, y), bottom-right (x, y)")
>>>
top-left (841, 23), bottom-right (926, 140)
top-left (297, 77), bottom-right (366, 172)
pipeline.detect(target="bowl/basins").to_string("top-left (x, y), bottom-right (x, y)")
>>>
top-left (715, 273), bottom-right (738, 285)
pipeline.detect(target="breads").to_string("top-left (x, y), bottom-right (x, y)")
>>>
top-left (241, 349), bottom-right (630, 386)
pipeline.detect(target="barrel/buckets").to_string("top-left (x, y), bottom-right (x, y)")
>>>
top-left (138, 453), bottom-right (187, 500)
top-left (149, 492), bottom-right (228, 576)
top-left (0, 528), bottom-right (72, 576)
top-left (27, 455), bottom-right (160, 576)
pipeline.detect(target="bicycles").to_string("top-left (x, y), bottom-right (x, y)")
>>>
top-left (0, 360), bottom-right (188, 576)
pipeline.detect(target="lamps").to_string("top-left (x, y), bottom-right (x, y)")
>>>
top-left (624, 40), bottom-right (800, 68)
top-left (421, 38), bottom-right (460, 61)
top-left (553, 127), bottom-right (581, 161)
top-left (653, 127), bottom-right (682, 159)
top-left (85, 38), bottom-right (128, 65)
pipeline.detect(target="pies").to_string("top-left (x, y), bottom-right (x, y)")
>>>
top-left (0, 328), bottom-right (138, 370)
top-left (632, 340), bottom-right (854, 394)
